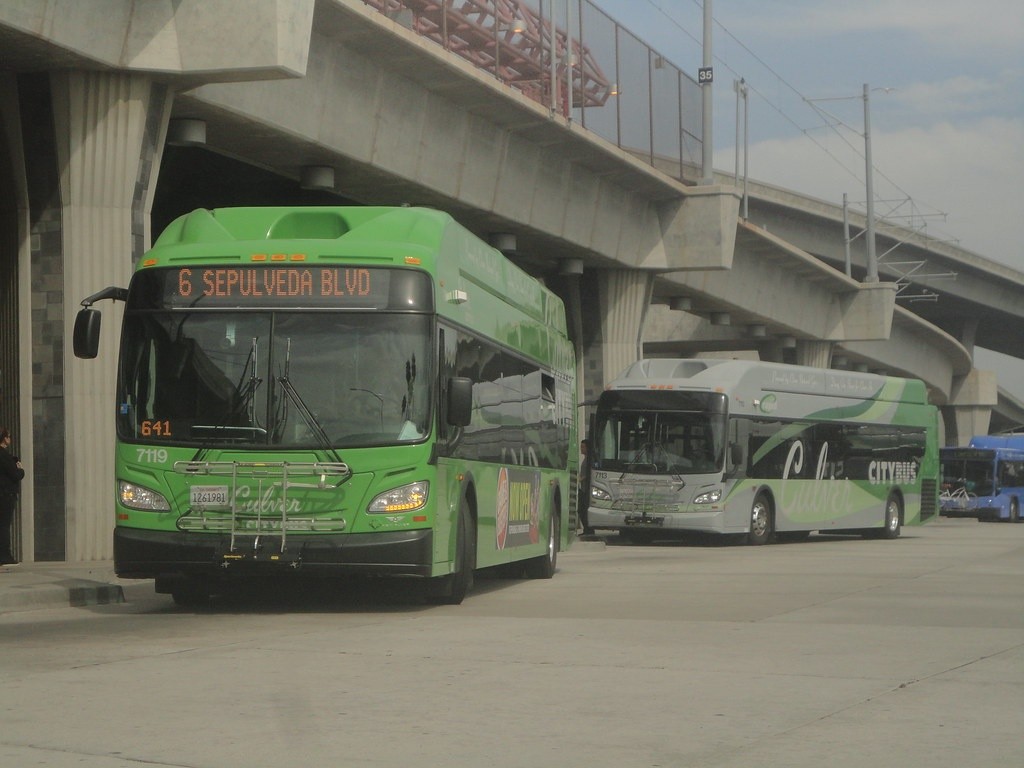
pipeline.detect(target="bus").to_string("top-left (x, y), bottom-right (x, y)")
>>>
top-left (72, 203), bottom-right (581, 606)
top-left (588, 355), bottom-right (943, 547)
top-left (938, 431), bottom-right (1023, 525)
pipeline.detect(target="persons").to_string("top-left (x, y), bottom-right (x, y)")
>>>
top-left (578, 439), bottom-right (594, 535)
top-left (321, 373), bottom-right (398, 420)
top-left (0, 427), bottom-right (24, 565)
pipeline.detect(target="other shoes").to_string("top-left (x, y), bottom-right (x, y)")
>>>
top-left (578, 529), bottom-right (595, 536)
